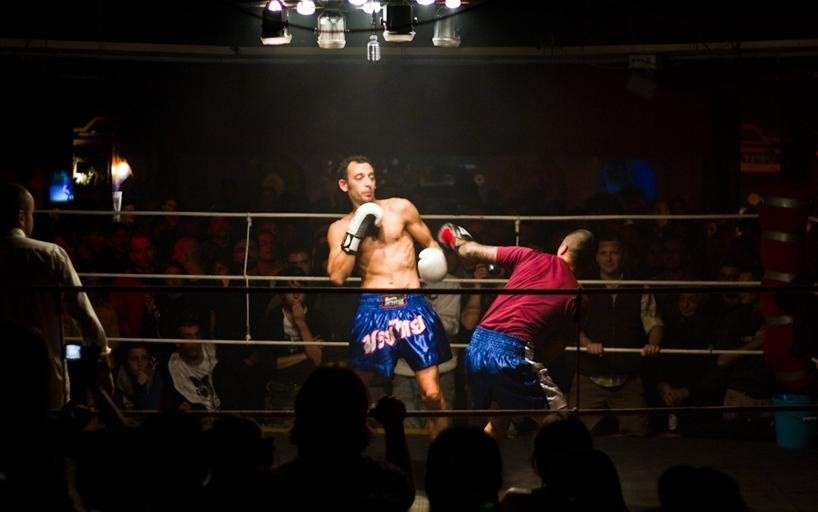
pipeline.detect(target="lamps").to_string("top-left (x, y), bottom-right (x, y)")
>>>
top-left (260, 0), bottom-right (463, 61)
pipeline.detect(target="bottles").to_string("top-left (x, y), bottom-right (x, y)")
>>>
top-left (667, 405), bottom-right (679, 433)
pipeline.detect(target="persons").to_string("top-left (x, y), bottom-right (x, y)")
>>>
top-left (325, 154), bottom-right (452, 450)
top-left (441, 212), bottom-right (596, 461)
top-left (391, 270), bottom-right (462, 429)
top-left (0, 158), bottom-right (321, 512)
top-left (657, 464), bottom-right (744, 512)
top-left (579, 125), bottom-right (778, 433)
top-left (276, 363), bottom-right (415, 510)
top-left (421, 418), bottom-right (627, 512)
top-left (451, 223), bottom-right (509, 328)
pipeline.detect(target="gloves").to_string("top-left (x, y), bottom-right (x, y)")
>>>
top-left (437, 222), bottom-right (474, 258)
top-left (417, 247), bottom-right (448, 283)
top-left (341, 201), bottom-right (384, 256)
